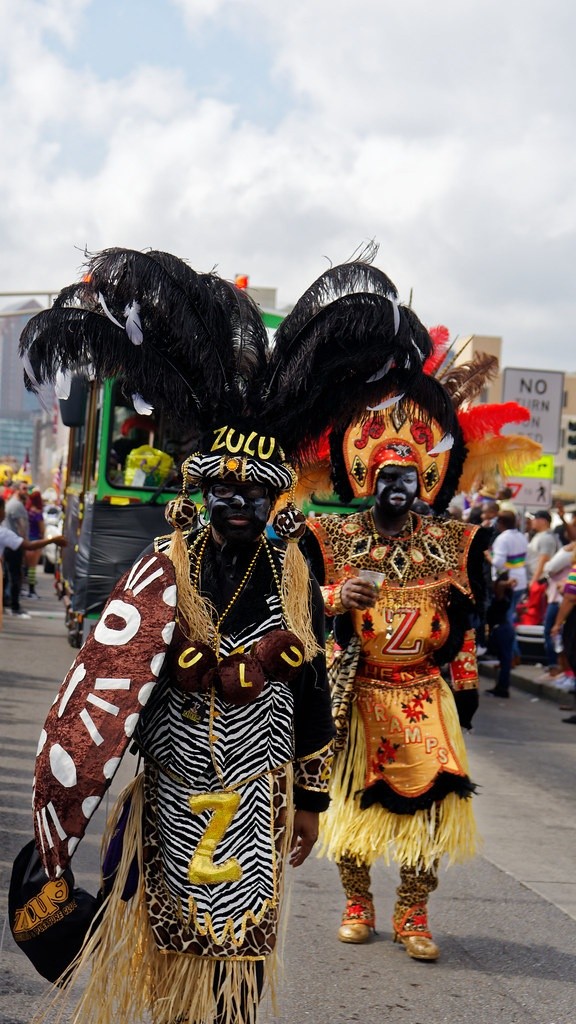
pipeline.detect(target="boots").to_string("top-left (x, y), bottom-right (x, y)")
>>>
top-left (391, 854), bottom-right (440, 960)
top-left (332, 849), bottom-right (380, 942)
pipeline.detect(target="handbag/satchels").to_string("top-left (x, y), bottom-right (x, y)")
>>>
top-left (124, 445), bottom-right (177, 488)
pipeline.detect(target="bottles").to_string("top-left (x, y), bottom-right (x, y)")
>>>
top-left (553, 634), bottom-right (564, 653)
top-left (491, 566), bottom-right (499, 581)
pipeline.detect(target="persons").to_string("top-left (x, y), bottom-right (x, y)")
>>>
top-left (8, 423), bottom-right (337, 1024)
top-left (0, 471), bottom-right (68, 627)
top-left (296, 445), bottom-right (493, 960)
top-left (447, 493), bottom-right (576, 725)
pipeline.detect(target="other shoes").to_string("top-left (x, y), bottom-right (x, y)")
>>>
top-left (495, 656), bottom-right (520, 669)
top-left (12, 611), bottom-right (32, 620)
top-left (532, 661), bottom-right (575, 722)
top-left (476, 646), bottom-right (487, 657)
top-left (484, 683), bottom-right (510, 699)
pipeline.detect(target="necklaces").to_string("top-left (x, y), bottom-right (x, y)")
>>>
top-left (370, 507), bottom-right (414, 611)
top-left (180, 526), bottom-right (286, 726)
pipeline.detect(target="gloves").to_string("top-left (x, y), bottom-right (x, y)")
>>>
top-left (454, 689), bottom-right (479, 731)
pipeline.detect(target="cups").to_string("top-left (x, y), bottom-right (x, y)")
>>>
top-left (359, 570), bottom-right (386, 605)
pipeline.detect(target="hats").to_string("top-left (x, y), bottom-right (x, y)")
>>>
top-left (479, 483), bottom-right (499, 499)
top-left (530, 511), bottom-right (551, 522)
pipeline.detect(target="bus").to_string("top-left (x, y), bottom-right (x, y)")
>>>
top-left (52, 312), bottom-right (380, 648)
